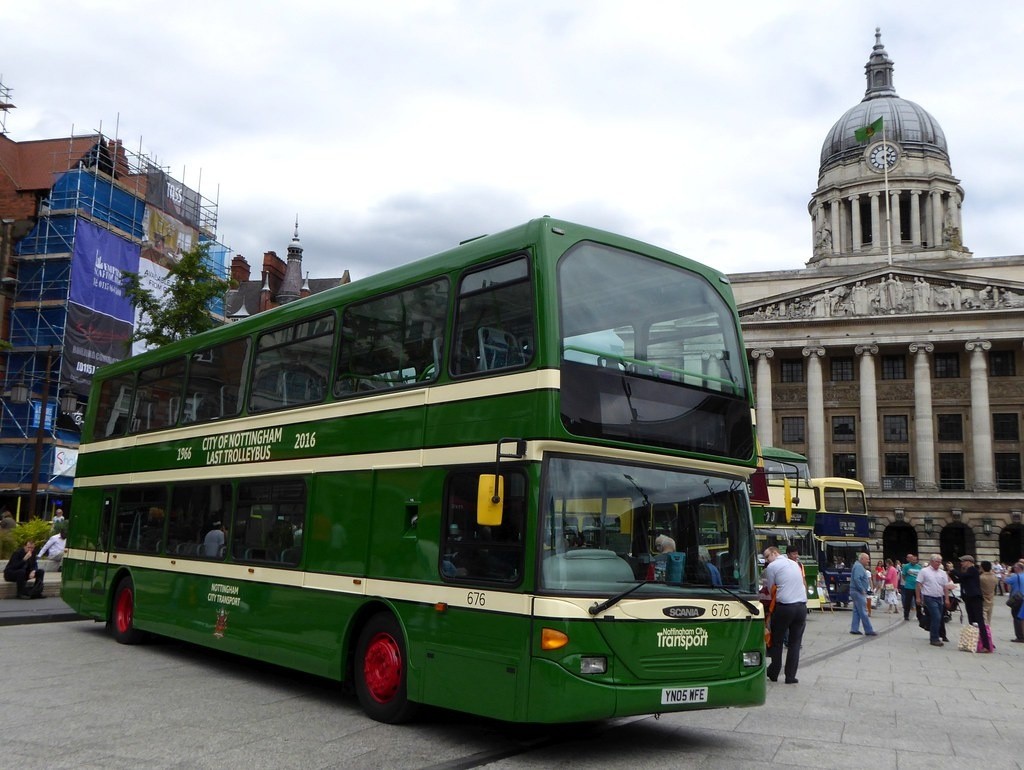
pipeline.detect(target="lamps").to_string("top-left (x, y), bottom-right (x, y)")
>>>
top-left (982, 518), bottom-right (992, 536)
top-left (869, 516), bottom-right (876, 534)
top-left (924, 518), bottom-right (933, 534)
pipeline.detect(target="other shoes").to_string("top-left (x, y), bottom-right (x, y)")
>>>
top-left (17, 595), bottom-right (30, 599)
top-left (849, 631), bottom-right (862, 635)
top-left (767, 672), bottom-right (777, 682)
top-left (930, 640), bottom-right (944, 646)
top-left (785, 679), bottom-right (798, 683)
top-left (865, 632), bottom-right (877, 636)
top-left (1006, 591), bottom-right (1024, 611)
top-left (905, 618), bottom-right (910, 620)
top-left (1011, 638), bottom-right (1023, 642)
top-left (29, 593), bottom-right (45, 599)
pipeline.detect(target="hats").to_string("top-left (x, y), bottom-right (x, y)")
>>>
top-left (958, 555), bottom-right (974, 562)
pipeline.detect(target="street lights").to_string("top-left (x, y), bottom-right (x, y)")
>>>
top-left (11, 370), bottom-right (77, 523)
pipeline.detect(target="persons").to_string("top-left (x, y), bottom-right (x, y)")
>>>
top-left (564, 536), bottom-right (583, 547)
top-left (760, 545), bottom-right (808, 684)
top-left (946, 555), bottom-right (996, 654)
top-left (36, 527), bottom-right (68, 572)
top-left (4, 537), bottom-right (48, 600)
top-left (1001, 559), bottom-right (1024, 643)
top-left (849, 553), bottom-right (879, 636)
top-left (991, 560), bottom-right (1013, 596)
top-left (203, 521), bottom-right (230, 559)
top-left (234, 526), bottom-right (271, 561)
top-left (0, 511), bottom-right (16, 529)
top-left (51, 508), bottom-right (65, 530)
top-left (628, 534), bottom-right (723, 586)
top-left (875, 554), bottom-right (961, 647)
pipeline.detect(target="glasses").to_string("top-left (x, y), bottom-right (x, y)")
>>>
top-left (765, 552), bottom-right (776, 558)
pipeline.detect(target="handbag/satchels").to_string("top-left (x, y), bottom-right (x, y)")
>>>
top-left (977, 624), bottom-right (994, 652)
top-left (885, 583), bottom-right (894, 590)
top-left (957, 622), bottom-right (980, 653)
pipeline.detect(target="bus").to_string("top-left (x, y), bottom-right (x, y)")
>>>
top-left (549, 445), bottom-right (820, 599)
top-left (61, 215), bottom-right (801, 726)
top-left (769, 479), bottom-right (872, 603)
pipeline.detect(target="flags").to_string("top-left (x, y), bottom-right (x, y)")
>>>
top-left (854, 116), bottom-right (883, 142)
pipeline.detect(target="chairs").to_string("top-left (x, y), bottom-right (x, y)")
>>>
top-left (157, 539), bottom-right (303, 563)
top-left (146, 328), bottom-right (661, 430)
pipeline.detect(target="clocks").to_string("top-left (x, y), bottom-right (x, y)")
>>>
top-left (869, 144), bottom-right (898, 169)
